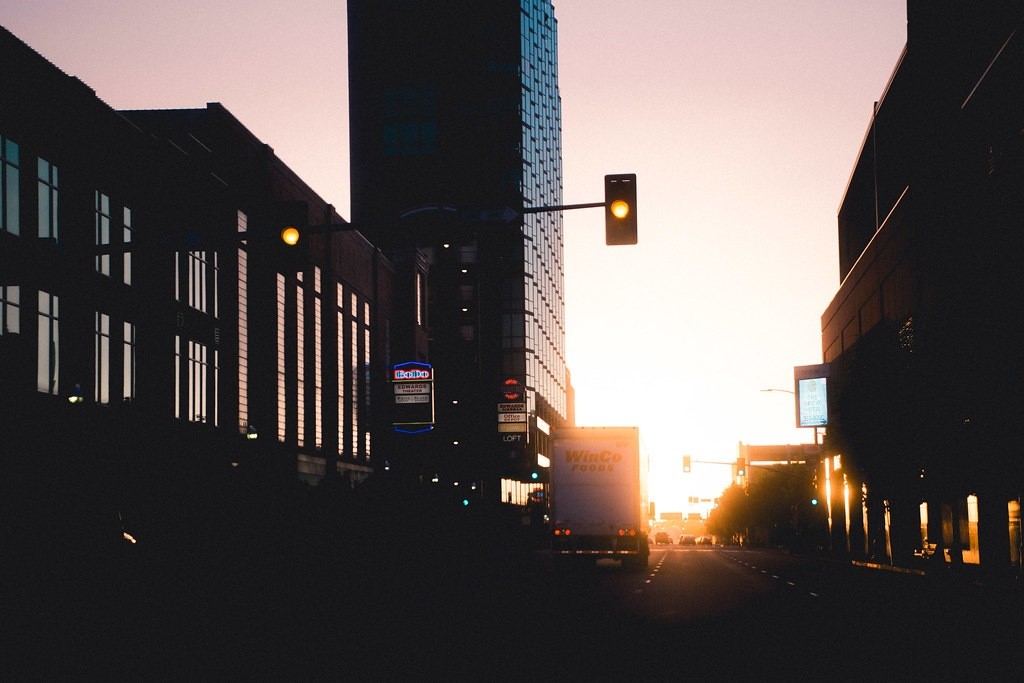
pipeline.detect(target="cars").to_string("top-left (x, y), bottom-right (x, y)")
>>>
top-left (657, 532), bottom-right (714, 546)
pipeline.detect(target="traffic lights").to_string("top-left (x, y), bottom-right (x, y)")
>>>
top-left (738, 457), bottom-right (746, 477)
top-left (273, 200), bottom-right (308, 261)
top-left (602, 173), bottom-right (637, 247)
top-left (682, 456), bottom-right (692, 472)
top-left (811, 498), bottom-right (818, 506)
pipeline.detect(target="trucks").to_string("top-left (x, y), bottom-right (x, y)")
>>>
top-left (550, 431), bottom-right (652, 570)
